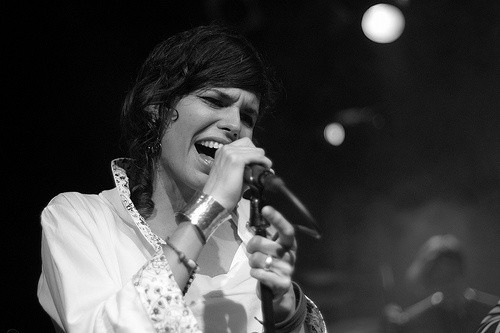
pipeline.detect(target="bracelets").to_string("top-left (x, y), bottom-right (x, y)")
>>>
top-left (166, 239), bottom-right (198, 272)
top-left (176, 190), bottom-right (232, 245)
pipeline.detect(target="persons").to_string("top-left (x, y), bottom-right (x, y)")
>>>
top-left (35, 21), bottom-right (328, 332)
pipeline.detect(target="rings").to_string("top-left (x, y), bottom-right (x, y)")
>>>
top-left (265, 255), bottom-right (273, 270)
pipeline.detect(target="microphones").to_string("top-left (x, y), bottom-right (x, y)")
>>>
top-left (245, 163), bottom-right (285, 193)
